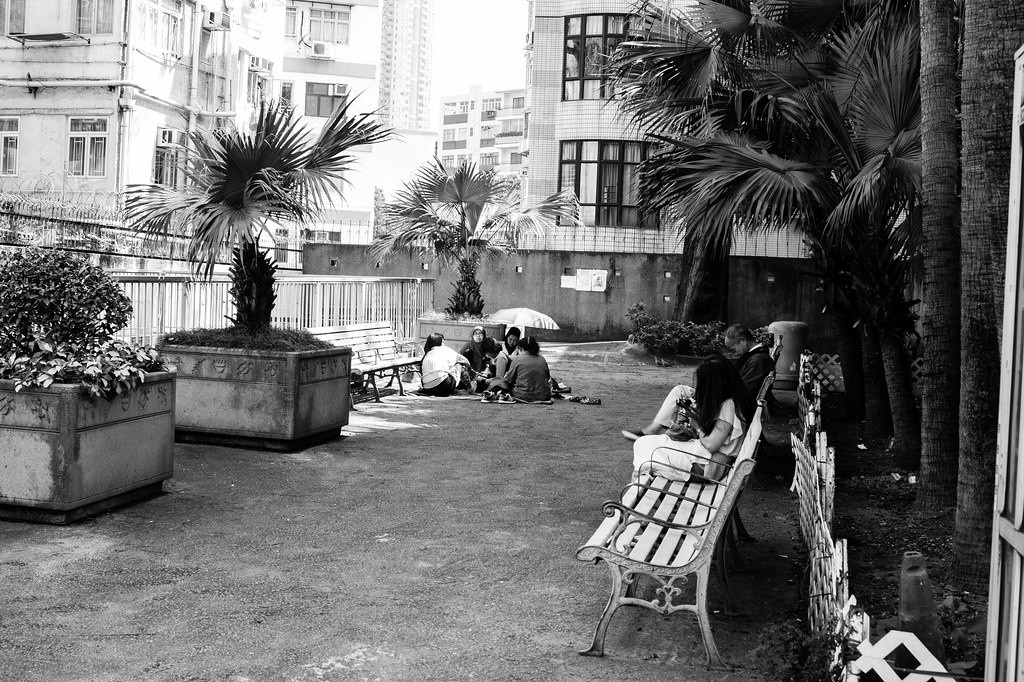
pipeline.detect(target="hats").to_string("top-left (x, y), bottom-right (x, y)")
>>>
top-left (469, 325), bottom-right (486, 339)
top-left (504, 327), bottom-right (521, 340)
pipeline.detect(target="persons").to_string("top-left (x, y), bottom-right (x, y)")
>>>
top-left (503, 337), bottom-right (552, 401)
top-left (471, 337), bottom-right (516, 394)
top-left (622, 323), bottom-right (776, 441)
top-left (421, 333), bottom-right (470, 396)
top-left (460, 326), bottom-right (491, 374)
top-left (631, 355), bottom-right (757, 485)
top-left (489, 327), bottom-right (521, 378)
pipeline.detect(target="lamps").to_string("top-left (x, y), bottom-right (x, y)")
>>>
top-left (119, 86), bottom-right (136, 111)
top-left (202, 10), bottom-right (230, 31)
top-left (333, 85), bottom-right (347, 96)
top-left (312, 42), bottom-right (332, 57)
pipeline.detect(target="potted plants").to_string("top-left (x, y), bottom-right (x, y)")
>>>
top-left (0, 246), bottom-right (176, 527)
top-left (363, 155), bottom-right (591, 355)
top-left (117, 86), bottom-right (406, 454)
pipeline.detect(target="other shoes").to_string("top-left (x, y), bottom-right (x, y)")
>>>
top-left (620, 429), bottom-right (642, 441)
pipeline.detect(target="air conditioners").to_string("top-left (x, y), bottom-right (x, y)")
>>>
top-left (310, 232), bottom-right (328, 240)
top-left (525, 32), bottom-right (532, 46)
top-left (156, 129), bottom-right (187, 149)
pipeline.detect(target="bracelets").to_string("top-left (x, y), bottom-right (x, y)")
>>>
top-left (696, 428), bottom-right (702, 436)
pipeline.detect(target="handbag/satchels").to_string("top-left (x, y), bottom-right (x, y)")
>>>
top-left (666, 414), bottom-right (700, 442)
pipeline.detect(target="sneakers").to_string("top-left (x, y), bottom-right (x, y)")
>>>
top-left (481, 391), bottom-right (498, 402)
top-left (497, 390), bottom-right (517, 404)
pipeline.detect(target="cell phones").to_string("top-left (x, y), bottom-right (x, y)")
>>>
top-left (680, 413), bottom-right (689, 418)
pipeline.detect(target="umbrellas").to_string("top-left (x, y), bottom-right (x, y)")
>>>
top-left (488, 308), bottom-right (560, 330)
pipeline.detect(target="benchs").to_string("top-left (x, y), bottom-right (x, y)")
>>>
top-left (305, 319), bottom-right (425, 411)
top-left (574, 334), bottom-right (783, 673)
